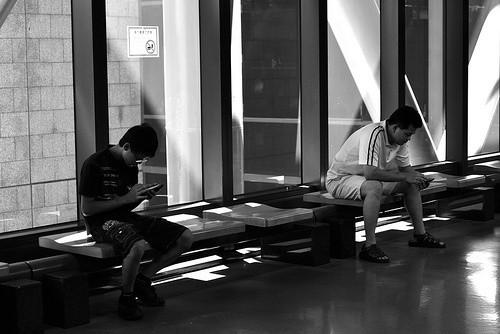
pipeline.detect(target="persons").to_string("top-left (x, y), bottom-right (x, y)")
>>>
top-left (324, 106), bottom-right (446, 263)
top-left (78, 123), bottom-right (193, 321)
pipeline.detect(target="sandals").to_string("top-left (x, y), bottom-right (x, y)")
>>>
top-left (408, 232), bottom-right (447, 248)
top-left (362, 244), bottom-right (389, 261)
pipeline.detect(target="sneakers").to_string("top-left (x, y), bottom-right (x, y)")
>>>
top-left (133, 281), bottom-right (165, 306)
top-left (118, 296), bottom-right (144, 321)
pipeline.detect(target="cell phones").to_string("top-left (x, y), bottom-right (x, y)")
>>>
top-left (424, 178), bottom-right (435, 182)
top-left (141, 183), bottom-right (164, 196)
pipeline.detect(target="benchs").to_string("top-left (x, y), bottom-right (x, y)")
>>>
top-left (0, 158), bottom-right (500, 334)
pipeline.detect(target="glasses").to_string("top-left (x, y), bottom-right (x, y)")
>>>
top-left (133, 152), bottom-right (149, 164)
